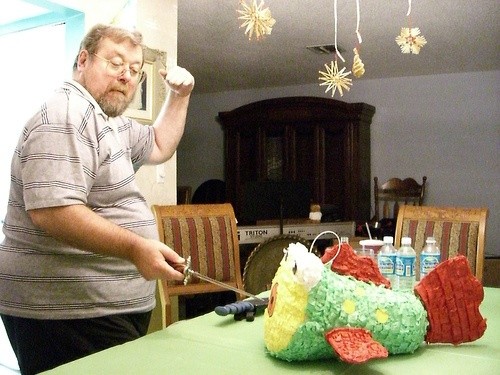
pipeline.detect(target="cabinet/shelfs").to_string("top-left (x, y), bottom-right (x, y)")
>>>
top-left (215, 96), bottom-right (376, 236)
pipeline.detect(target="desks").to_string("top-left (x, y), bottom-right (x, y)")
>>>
top-left (37, 279), bottom-right (500, 375)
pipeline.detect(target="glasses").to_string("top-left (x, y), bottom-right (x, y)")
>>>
top-left (87, 50), bottom-right (146, 86)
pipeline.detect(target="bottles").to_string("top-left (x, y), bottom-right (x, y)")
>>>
top-left (378, 236), bottom-right (398, 287)
top-left (419, 236), bottom-right (441, 286)
top-left (395, 237), bottom-right (417, 290)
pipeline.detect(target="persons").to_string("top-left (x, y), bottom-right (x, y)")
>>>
top-left (0, 22), bottom-right (195, 375)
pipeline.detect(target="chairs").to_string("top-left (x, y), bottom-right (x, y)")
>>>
top-left (374, 176), bottom-right (427, 220)
top-left (394, 205), bottom-right (489, 285)
top-left (151, 202), bottom-right (245, 330)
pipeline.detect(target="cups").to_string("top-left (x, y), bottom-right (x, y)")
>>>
top-left (359, 239), bottom-right (384, 258)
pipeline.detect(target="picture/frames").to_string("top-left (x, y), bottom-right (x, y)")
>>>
top-left (122, 44), bottom-right (167, 126)
